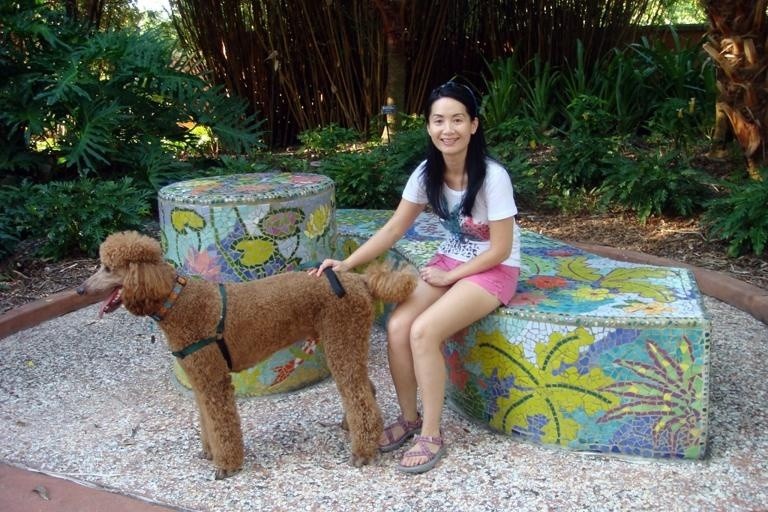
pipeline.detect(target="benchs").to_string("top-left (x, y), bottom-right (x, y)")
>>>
top-left (335, 208), bottom-right (713, 459)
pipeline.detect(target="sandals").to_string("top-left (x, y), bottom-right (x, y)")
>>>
top-left (376, 412), bottom-right (423, 452)
top-left (398, 430), bottom-right (447, 473)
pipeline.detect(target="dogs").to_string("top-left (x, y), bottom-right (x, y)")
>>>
top-left (76, 229), bottom-right (418, 480)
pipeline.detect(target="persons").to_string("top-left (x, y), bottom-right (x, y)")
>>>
top-left (307, 79), bottom-right (521, 474)
